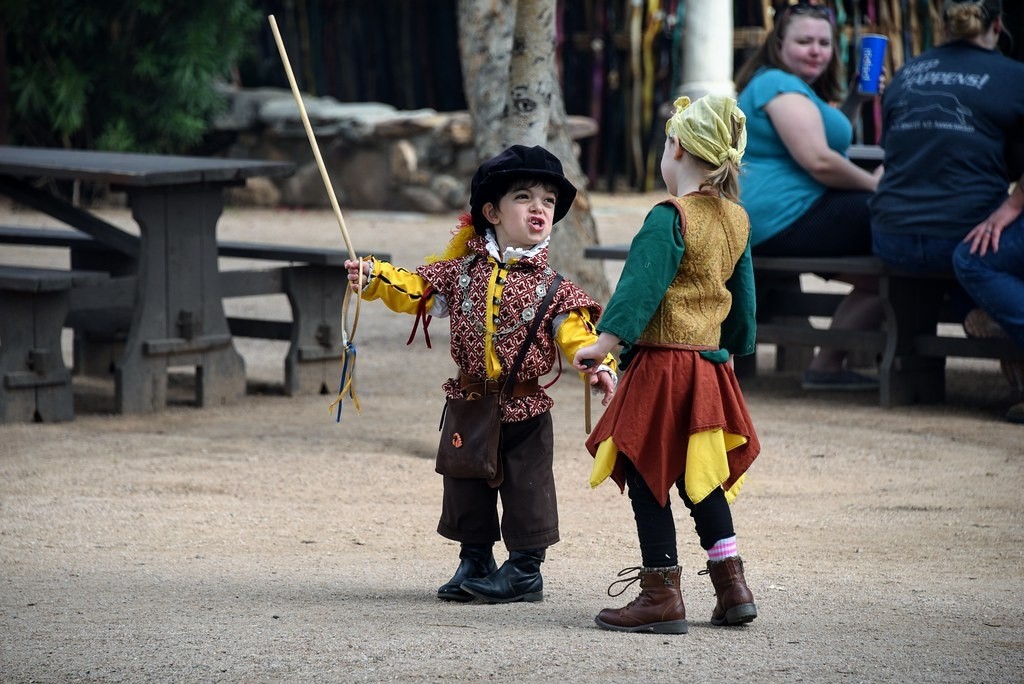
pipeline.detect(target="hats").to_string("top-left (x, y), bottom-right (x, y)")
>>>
top-left (470, 145), bottom-right (577, 226)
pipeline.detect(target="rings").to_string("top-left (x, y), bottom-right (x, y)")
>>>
top-left (985, 229), bottom-right (993, 233)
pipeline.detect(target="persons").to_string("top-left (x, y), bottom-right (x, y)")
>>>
top-left (730, 3), bottom-right (888, 391)
top-left (865, 0), bottom-right (1024, 399)
top-left (573, 91), bottom-right (760, 636)
top-left (951, 177), bottom-right (1024, 423)
top-left (342, 145), bottom-right (618, 607)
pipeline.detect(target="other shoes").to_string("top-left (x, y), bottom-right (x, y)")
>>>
top-left (802, 368), bottom-right (878, 389)
top-left (1007, 403), bottom-right (1024, 424)
top-left (964, 308), bottom-right (1008, 339)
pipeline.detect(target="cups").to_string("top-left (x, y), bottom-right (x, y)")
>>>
top-left (856, 33), bottom-right (889, 96)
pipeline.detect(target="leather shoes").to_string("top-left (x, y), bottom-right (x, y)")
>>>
top-left (594, 567), bottom-right (689, 635)
top-left (460, 550), bottom-right (546, 602)
top-left (698, 555), bottom-right (757, 625)
top-left (437, 550), bottom-right (499, 601)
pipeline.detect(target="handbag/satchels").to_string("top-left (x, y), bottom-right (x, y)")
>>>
top-left (435, 396), bottom-right (503, 480)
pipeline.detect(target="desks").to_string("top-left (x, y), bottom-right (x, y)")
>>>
top-left (0, 145), bottom-right (298, 415)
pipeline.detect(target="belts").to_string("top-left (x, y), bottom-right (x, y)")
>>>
top-left (459, 369), bottom-right (539, 399)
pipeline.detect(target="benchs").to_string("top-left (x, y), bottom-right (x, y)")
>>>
top-left (0, 227), bottom-right (391, 426)
top-left (583, 245), bottom-right (1024, 410)
